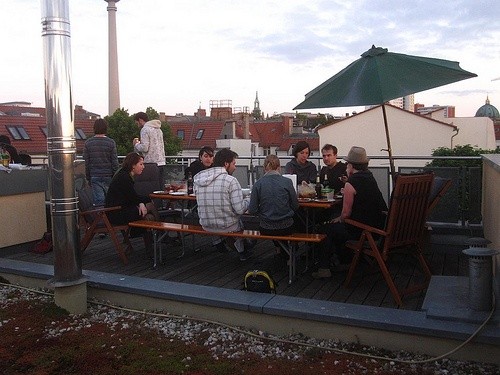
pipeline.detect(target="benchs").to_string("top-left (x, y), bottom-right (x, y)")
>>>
top-left (129, 220), bottom-right (327, 287)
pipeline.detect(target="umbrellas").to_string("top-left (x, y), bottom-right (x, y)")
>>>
top-left (293, 43), bottom-right (479, 204)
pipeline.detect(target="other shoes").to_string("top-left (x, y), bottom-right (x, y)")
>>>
top-left (312, 269), bottom-right (331, 279)
top-left (331, 260), bottom-right (350, 271)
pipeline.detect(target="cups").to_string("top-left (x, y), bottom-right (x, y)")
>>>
top-left (164, 184), bottom-right (170, 194)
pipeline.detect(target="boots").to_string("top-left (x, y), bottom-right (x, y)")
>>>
top-left (144, 232), bottom-right (159, 260)
top-left (160, 229), bottom-right (182, 245)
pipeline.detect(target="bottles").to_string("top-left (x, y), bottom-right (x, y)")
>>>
top-left (315, 175), bottom-right (322, 199)
top-left (323, 173), bottom-right (330, 188)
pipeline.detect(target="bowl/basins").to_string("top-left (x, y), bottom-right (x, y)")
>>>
top-left (242, 188), bottom-right (250, 197)
top-left (321, 188), bottom-right (334, 201)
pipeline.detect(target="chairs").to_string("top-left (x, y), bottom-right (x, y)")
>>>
top-left (79, 163), bottom-right (182, 264)
top-left (344, 170), bottom-right (453, 306)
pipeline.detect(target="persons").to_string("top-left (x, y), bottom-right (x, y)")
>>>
top-left (83, 116), bottom-right (120, 238)
top-left (317, 144), bottom-right (348, 200)
top-left (284, 141), bottom-right (318, 229)
top-left (131, 112), bottom-right (166, 207)
top-left (188, 149), bottom-right (260, 262)
top-left (0, 135), bottom-right (18, 163)
top-left (249, 152), bottom-right (307, 257)
top-left (188, 146), bottom-right (218, 202)
top-left (308, 144), bottom-right (389, 279)
top-left (106, 149), bottom-right (182, 260)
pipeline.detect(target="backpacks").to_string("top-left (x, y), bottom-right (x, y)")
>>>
top-left (241, 269), bottom-right (278, 294)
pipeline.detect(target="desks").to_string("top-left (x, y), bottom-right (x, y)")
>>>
top-left (149, 193), bottom-right (342, 271)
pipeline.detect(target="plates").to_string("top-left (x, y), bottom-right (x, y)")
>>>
top-left (312, 198), bottom-right (335, 202)
top-left (169, 192), bottom-right (187, 195)
top-left (153, 190), bottom-right (169, 194)
